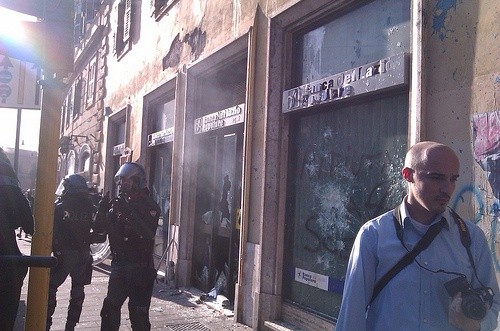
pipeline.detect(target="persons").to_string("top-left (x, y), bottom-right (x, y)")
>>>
top-left (336, 141), bottom-right (500, 331)
top-left (46, 175), bottom-right (93, 331)
top-left (93, 162), bottom-right (161, 331)
top-left (0, 149), bottom-right (34, 331)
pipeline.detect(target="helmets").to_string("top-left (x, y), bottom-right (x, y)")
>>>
top-left (120, 162), bottom-right (146, 190)
top-left (64, 173), bottom-right (89, 195)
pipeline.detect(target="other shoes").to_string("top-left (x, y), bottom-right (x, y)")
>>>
top-left (17, 232), bottom-right (22, 239)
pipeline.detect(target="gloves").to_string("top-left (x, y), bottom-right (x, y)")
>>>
top-left (93, 196), bottom-right (114, 214)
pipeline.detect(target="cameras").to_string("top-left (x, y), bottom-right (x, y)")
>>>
top-left (445, 277), bottom-right (495, 320)
